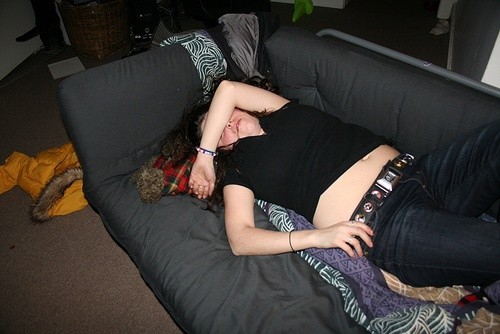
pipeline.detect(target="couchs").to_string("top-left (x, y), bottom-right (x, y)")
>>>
top-left (54, 14), bottom-right (500, 334)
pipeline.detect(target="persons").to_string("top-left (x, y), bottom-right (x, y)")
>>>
top-left (181, 79), bottom-right (500, 289)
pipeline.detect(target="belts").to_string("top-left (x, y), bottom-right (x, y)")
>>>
top-left (345, 151), bottom-right (416, 252)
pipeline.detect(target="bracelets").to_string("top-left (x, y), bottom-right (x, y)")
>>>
top-left (196, 146), bottom-right (218, 157)
top-left (288, 229), bottom-right (298, 252)
